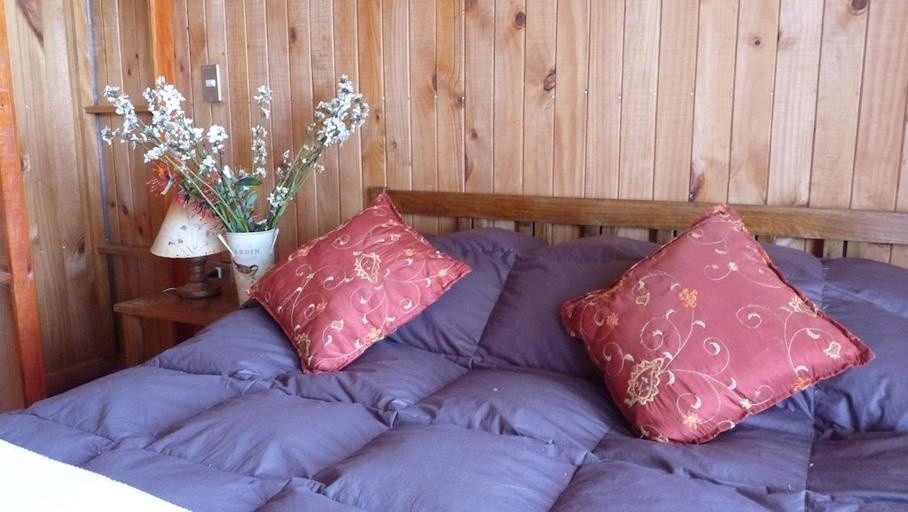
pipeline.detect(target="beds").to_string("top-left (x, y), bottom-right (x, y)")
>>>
top-left (0, 187), bottom-right (905, 511)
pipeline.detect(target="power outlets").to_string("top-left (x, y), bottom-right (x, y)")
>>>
top-left (208, 260), bottom-right (232, 287)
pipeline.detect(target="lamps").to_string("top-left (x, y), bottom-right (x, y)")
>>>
top-left (148, 194), bottom-right (223, 300)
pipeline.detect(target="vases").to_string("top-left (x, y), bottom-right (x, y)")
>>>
top-left (214, 229), bottom-right (279, 312)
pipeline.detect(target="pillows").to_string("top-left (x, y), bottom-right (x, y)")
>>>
top-left (246, 191), bottom-right (474, 375)
top-left (556, 202), bottom-right (878, 445)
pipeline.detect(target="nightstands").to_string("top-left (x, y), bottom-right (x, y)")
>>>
top-left (114, 284), bottom-right (237, 369)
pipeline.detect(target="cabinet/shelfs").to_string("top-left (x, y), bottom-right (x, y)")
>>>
top-left (2, 2), bottom-right (114, 414)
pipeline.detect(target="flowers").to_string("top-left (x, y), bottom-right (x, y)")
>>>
top-left (100, 71), bottom-right (373, 231)
